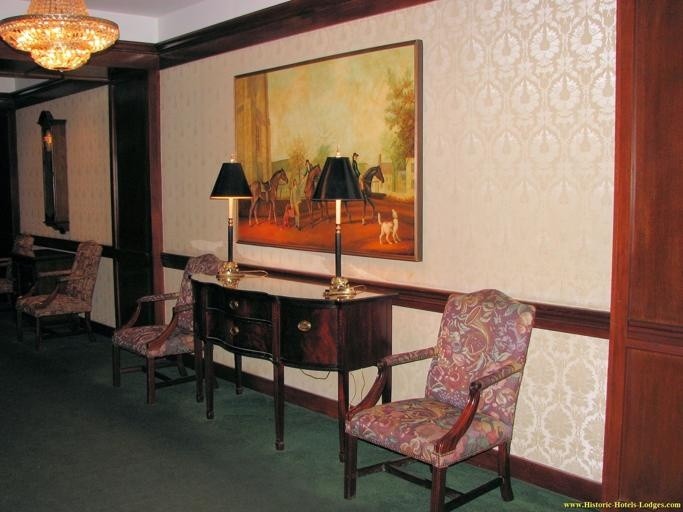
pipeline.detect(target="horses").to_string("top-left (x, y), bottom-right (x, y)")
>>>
top-left (249, 167), bottom-right (288, 227)
top-left (343, 165), bottom-right (384, 224)
top-left (304, 163), bottom-right (331, 229)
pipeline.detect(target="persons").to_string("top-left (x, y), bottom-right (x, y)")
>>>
top-left (303, 160), bottom-right (313, 178)
top-left (352, 153), bottom-right (364, 190)
top-left (289, 179), bottom-right (302, 231)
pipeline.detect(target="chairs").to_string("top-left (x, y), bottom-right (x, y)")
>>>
top-left (0, 235), bottom-right (227, 404)
top-left (339, 288), bottom-right (536, 511)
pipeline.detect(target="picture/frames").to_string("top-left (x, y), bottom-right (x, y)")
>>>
top-left (233, 40), bottom-right (422, 263)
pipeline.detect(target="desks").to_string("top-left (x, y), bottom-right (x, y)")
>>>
top-left (190, 268), bottom-right (399, 462)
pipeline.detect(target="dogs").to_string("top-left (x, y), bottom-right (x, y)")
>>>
top-left (378, 209), bottom-right (401, 245)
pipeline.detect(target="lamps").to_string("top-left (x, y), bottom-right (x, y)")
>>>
top-left (210, 145), bottom-right (361, 297)
top-left (0, 0), bottom-right (119, 73)
